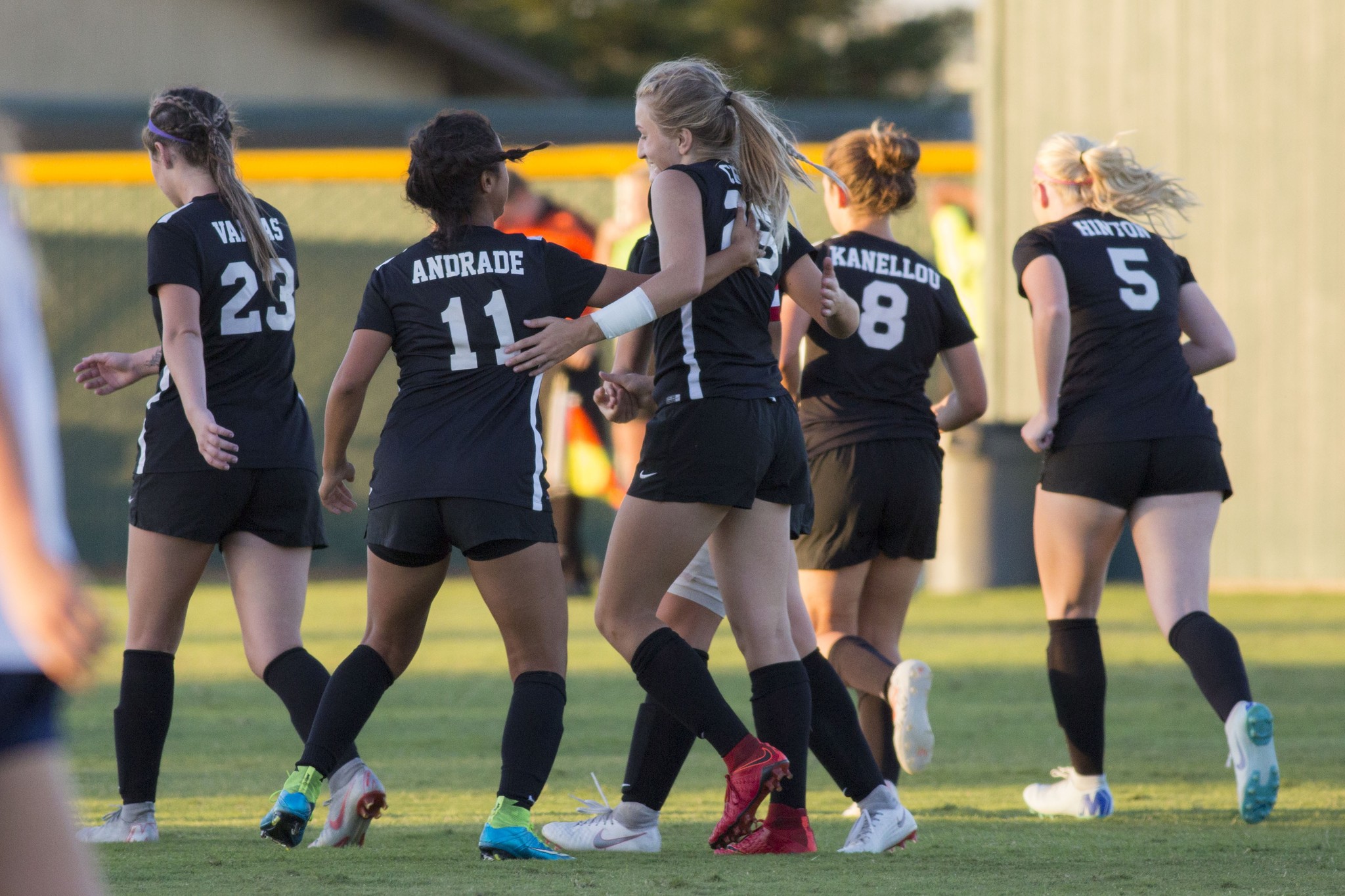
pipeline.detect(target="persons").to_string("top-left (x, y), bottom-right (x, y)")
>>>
top-left (254, 110), bottom-right (582, 864)
top-left (768, 118), bottom-right (989, 818)
top-left (535, 206), bottom-right (923, 859)
top-left (1, 113), bottom-right (124, 896)
top-left (75, 90), bottom-right (390, 846)
top-left (1010, 129), bottom-right (1285, 827)
top-left (475, 167), bottom-right (990, 602)
top-left (501, 56), bottom-right (821, 858)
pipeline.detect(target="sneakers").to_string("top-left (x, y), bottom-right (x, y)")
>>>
top-left (709, 736), bottom-right (793, 850)
top-left (714, 801), bottom-right (818, 854)
top-left (842, 779), bottom-right (899, 815)
top-left (541, 800), bottom-right (662, 854)
top-left (306, 757), bottom-right (389, 850)
top-left (887, 659), bottom-right (935, 775)
top-left (478, 796), bottom-right (577, 863)
top-left (75, 801), bottom-right (160, 845)
top-left (842, 784), bottom-right (918, 854)
top-left (1022, 781), bottom-right (1112, 821)
top-left (259, 764), bottom-right (324, 851)
top-left (1223, 700), bottom-right (1281, 824)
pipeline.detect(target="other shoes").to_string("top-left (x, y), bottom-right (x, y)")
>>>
top-left (563, 564), bottom-right (590, 597)
